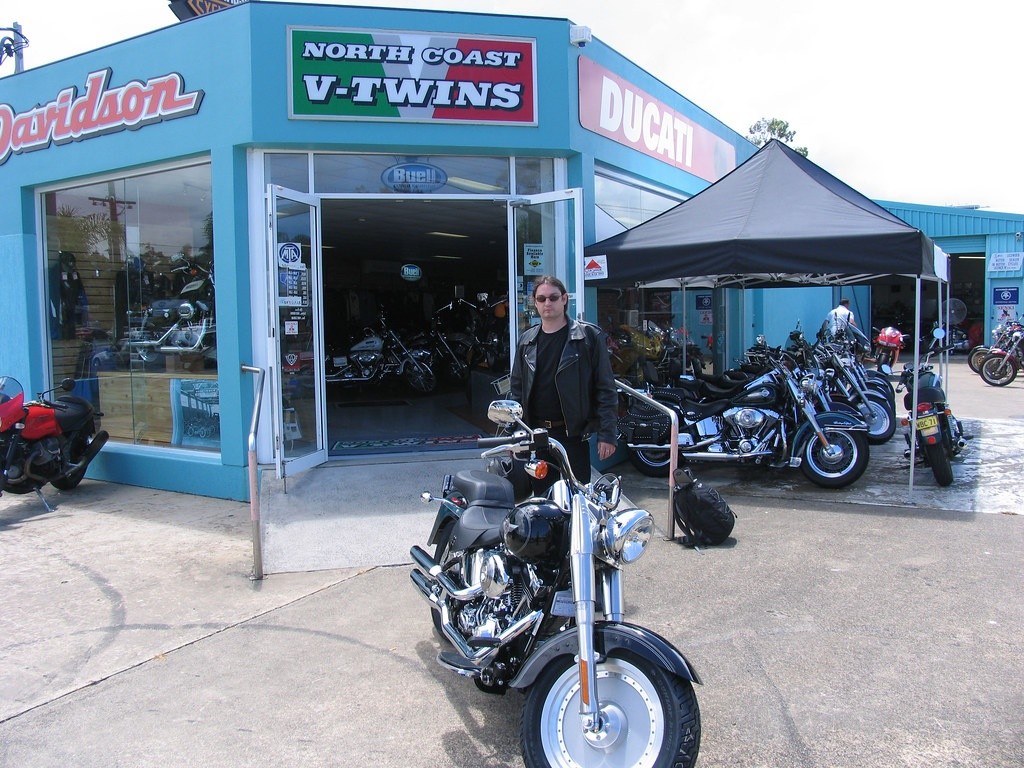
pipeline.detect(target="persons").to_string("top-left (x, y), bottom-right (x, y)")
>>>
top-left (504, 271), bottom-right (619, 504)
top-left (820, 296), bottom-right (858, 345)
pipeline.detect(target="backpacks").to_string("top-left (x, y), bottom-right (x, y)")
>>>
top-left (673, 468), bottom-right (737, 547)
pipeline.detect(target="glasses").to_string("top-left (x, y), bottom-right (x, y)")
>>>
top-left (535, 293), bottom-right (564, 302)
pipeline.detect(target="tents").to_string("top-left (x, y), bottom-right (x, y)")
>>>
top-left (583, 135), bottom-right (952, 502)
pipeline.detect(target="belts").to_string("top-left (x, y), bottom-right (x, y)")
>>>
top-left (533, 420), bottom-right (564, 428)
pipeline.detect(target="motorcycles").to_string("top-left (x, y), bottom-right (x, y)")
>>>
top-left (88, 251), bottom-right (912, 490)
top-left (880, 324), bottom-right (974, 487)
top-left (0, 372), bottom-right (109, 511)
top-left (979, 312), bottom-right (1024, 387)
top-left (963, 309), bottom-right (1024, 374)
top-left (404, 394), bottom-right (706, 768)
